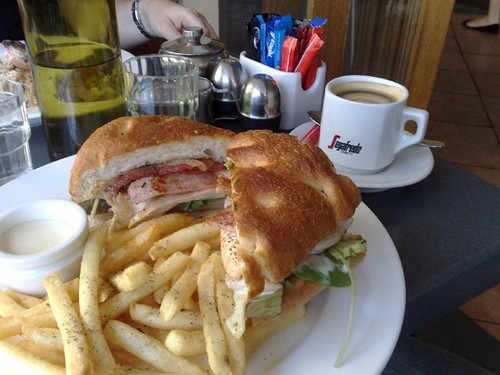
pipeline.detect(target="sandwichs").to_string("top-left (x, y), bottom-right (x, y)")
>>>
top-left (67, 114), bottom-right (239, 229)
top-left (219, 125), bottom-right (368, 341)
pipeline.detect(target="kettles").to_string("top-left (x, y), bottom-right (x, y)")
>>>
top-left (154, 26), bottom-right (231, 79)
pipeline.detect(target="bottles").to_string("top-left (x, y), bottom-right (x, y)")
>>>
top-left (18, 0), bottom-right (122, 160)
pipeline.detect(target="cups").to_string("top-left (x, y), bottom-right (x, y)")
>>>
top-left (120, 55), bottom-right (211, 122)
top-left (0, 74), bottom-right (33, 150)
top-left (325, 75), bottom-right (428, 172)
top-left (1, 198), bottom-right (87, 296)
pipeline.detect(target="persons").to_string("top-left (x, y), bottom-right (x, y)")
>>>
top-left (462, 0), bottom-right (500, 34)
top-left (58, 0), bottom-right (219, 50)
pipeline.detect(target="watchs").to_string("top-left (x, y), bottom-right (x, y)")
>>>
top-left (132, 0), bottom-right (156, 41)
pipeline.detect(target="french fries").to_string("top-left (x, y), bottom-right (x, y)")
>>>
top-left (0, 205), bottom-right (369, 375)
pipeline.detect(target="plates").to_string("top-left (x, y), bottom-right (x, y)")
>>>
top-left (0, 152), bottom-right (406, 371)
top-left (17, 37), bottom-right (148, 125)
top-left (287, 117), bottom-right (439, 193)
